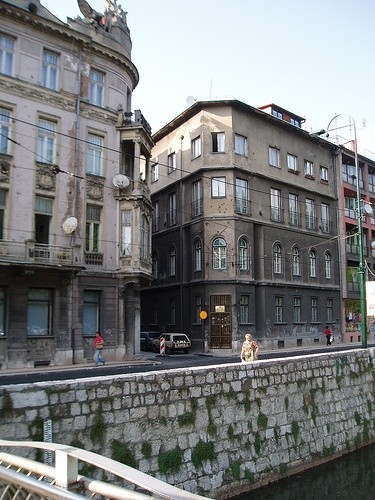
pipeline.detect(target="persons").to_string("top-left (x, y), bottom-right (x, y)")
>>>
top-left (241, 334), bottom-right (260, 361)
top-left (94, 332), bottom-right (105, 367)
top-left (324, 326), bottom-right (332, 346)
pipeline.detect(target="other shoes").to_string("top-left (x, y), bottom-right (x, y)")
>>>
top-left (329, 343), bottom-right (331, 345)
top-left (327, 345), bottom-right (329, 346)
top-left (95, 364), bottom-right (98, 367)
top-left (103, 360), bottom-right (105, 365)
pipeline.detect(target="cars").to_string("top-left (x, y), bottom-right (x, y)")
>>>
top-left (141, 331), bottom-right (161, 352)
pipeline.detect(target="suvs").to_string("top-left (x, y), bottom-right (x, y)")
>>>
top-left (151, 332), bottom-right (192, 355)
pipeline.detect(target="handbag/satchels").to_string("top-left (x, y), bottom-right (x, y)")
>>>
top-left (330, 335), bottom-right (333, 343)
top-left (96, 342), bottom-right (103, 350)
